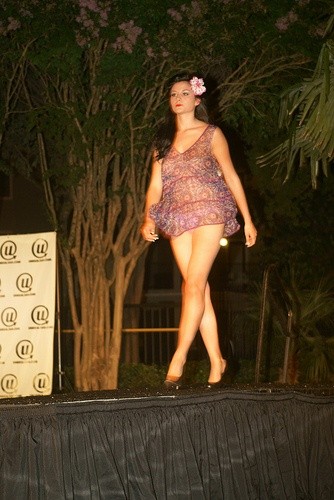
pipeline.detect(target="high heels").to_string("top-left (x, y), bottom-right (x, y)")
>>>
top-left (162, 364), bottom-right (191, 389)
top-left (208, 366), bottom-right (233, 390)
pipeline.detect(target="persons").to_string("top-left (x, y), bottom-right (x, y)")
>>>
top-left (140, 71), bottom-right (258, 391)
